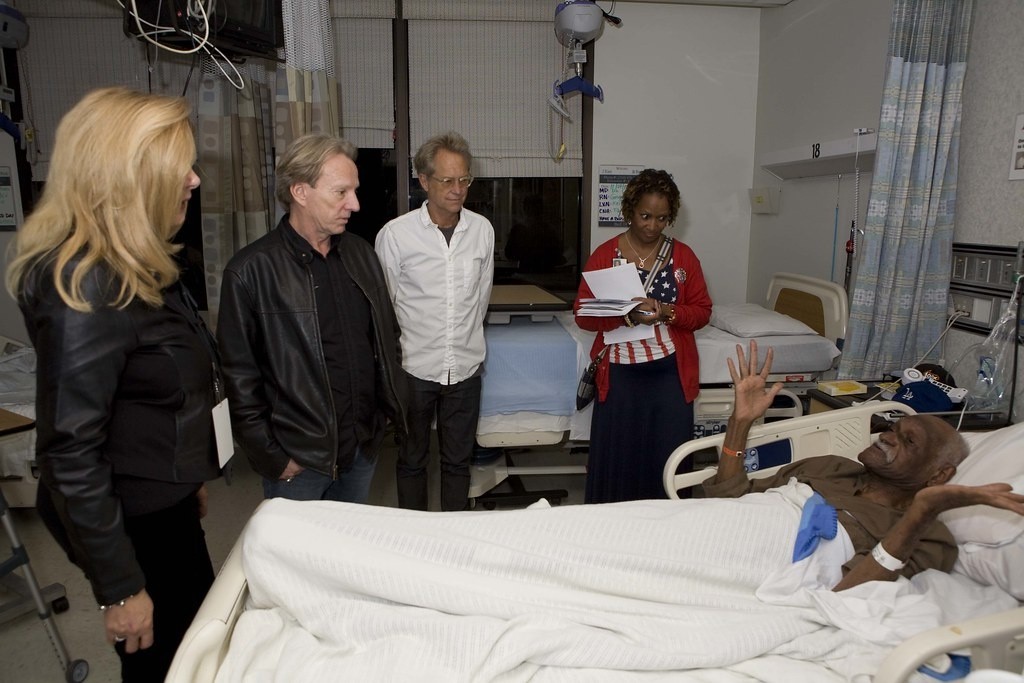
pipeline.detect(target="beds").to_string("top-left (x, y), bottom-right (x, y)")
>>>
top-left (467, 270), bottom-right (850, 505)
top-left (162, 401), bottom-right (1024, 683)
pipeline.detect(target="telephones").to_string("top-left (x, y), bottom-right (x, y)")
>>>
top-left (903, 368), bottom-right (969, 403)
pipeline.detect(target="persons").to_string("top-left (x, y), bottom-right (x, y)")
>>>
top-left (615, 261), bottom-right (620, 266)
top-left (374, 131), bottom-right (494, 512)
top-left (215, 133), bottom-right (408, 504)
top-left (5, 85), bottom-right (227, 683)
top-left (573, 169), bottom-right (713, 504)
top-left (701, 339), bottom-right (1024, 592)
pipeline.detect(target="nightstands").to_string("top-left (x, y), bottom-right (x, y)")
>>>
top-left (806, 377), bottom-right (1013, 433)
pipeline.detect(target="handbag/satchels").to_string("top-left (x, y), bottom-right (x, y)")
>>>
top-left (576, 362), bottom-right (598, 411)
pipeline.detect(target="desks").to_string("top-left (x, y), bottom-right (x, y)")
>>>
top-left (483, 283), bottom-right (574, 326)
top-left (0, 404), bottom-right (90, 683)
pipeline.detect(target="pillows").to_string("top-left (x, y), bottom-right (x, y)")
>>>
top-left (935, 420), bottom-right (1024, 602)
top-left (708, 302), bottom-right (820, 338)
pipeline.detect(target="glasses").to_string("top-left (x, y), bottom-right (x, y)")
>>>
top-left (424, 172), bottom-right (474, 189)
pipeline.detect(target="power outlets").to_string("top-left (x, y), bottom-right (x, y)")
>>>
top-left (950, 253), bottom-right (1017, 318)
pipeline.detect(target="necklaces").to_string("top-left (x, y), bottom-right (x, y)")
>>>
top-left (625, 232), bottom-right (661, 268)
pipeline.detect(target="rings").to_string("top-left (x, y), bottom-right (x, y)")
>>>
top-left (115, 636), bottom-right (124, 642)
top-left (287, 476), bottom-right (292, 483)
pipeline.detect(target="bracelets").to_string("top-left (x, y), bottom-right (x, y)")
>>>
top-left (99, 595), bottom-right (133, 610)
top-left (624, 315), bottom-right (640, 328)
top-left (871, 541), bottom-right (908, 572)
top-left (663, 309), bottom-right (676, 326)
top-left (722, 446), bottom-right (745, 457)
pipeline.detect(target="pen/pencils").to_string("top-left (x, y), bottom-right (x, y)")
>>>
top-left (635, 310), bottom-right (656, 316)
